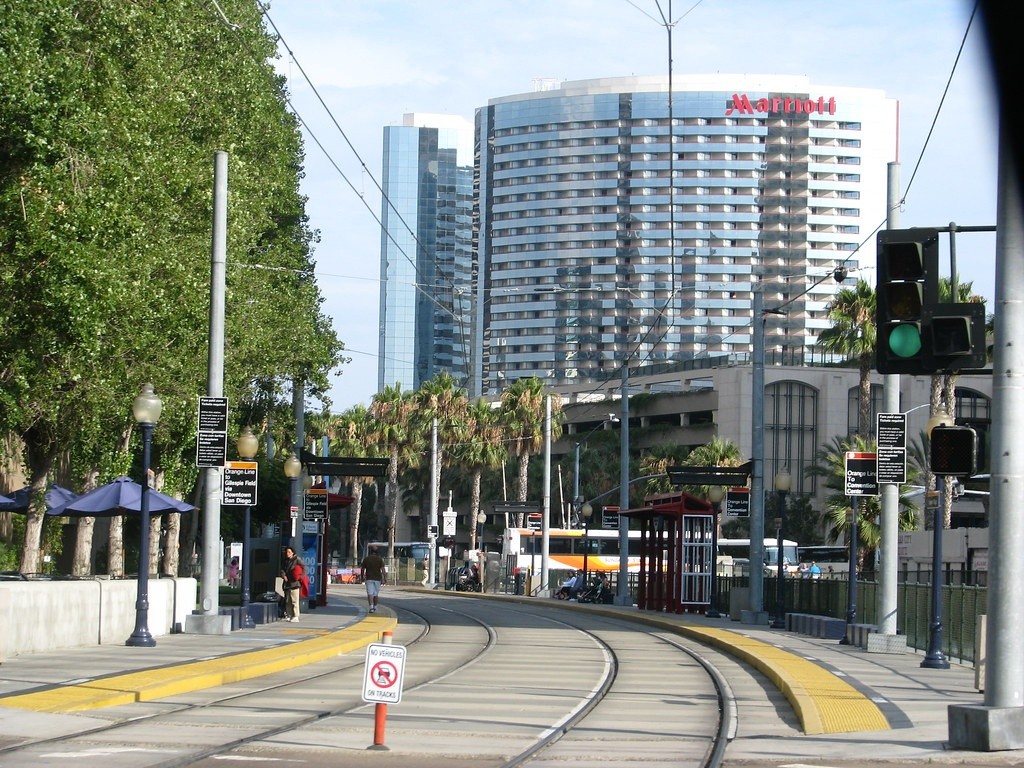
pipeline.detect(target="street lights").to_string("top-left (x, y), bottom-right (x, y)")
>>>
top-left (236, 424), bottom-right (256, 629)
top-left (839, 441), bottom-right (860, 647)
top-left (478, 509), bottom-right (486, 553)
top-left (582, 503), bottom-right (593, 589)
top-left (125, 382), bottom-right (157, 647)
top-left (920, 403), bottom-right (951, 669)
top-left (773, 466), bottom-right (793, 629)
top-left (705, 485), bottom-right (722, 618)
top-left (284, 452), bottom-right (301, 547)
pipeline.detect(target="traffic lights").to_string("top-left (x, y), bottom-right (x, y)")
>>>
top-left (931, 422), bottom-right (978, 476)
top-left (876, 228), bottom-right (986, 374)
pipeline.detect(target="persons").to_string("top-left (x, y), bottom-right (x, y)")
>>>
top-left (553, 570), bottom-right (576, 599)
top-left (799, 560), bottom-right (824, 579)
top-left (569, 569), bottom-right (585, 602)
top-left (577, 571), bottom-right (611, 603)
top-left (229, 558), bottom-right (239, 589)
top-left (421, 558), bottom-right (429, 585)
top-left (360, 545), bottom-right (386, 613)
top-left (281, 547), bottom-right (307, 622)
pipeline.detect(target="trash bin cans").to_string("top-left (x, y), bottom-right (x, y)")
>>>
top-left (336, 574), bottom-right (343, 584)
top-left (514, 572), bottom-right (526, 595)
top-left (20, 572), bottom-right (54, 580)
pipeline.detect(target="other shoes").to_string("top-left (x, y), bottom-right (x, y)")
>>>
top-left (569, 598), bottom-right (575, 601)
top-left (369, 608), bottom-right (376, 613)
top-left (287, 617), bottom-right (291, 622)
top-left (290, 617), bottom-right (300, 623)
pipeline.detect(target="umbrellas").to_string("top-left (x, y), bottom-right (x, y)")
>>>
top-left (0, 482), bottom-right (79, 515)
top-left (44, 475), bottom-right (199, 579)
top-left (0, 495), bottom-right (15, 504)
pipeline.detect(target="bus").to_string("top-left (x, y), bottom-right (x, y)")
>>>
top-left (502, 526), bottom-right (851, 592)
top-left (366, 542), bottom-right (429, 570)
top-left (873, 474), bottom-right (991, 585)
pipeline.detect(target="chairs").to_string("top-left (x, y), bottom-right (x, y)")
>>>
top-left (0, 573), bottom-right (138, 581)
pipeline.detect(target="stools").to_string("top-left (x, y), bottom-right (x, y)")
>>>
top-left (192, 601), bottom-right (279, 631)
top-left (785, 613), bottom-right (846, 638)
top-left (847, 624), bottom-right (902, 648)
